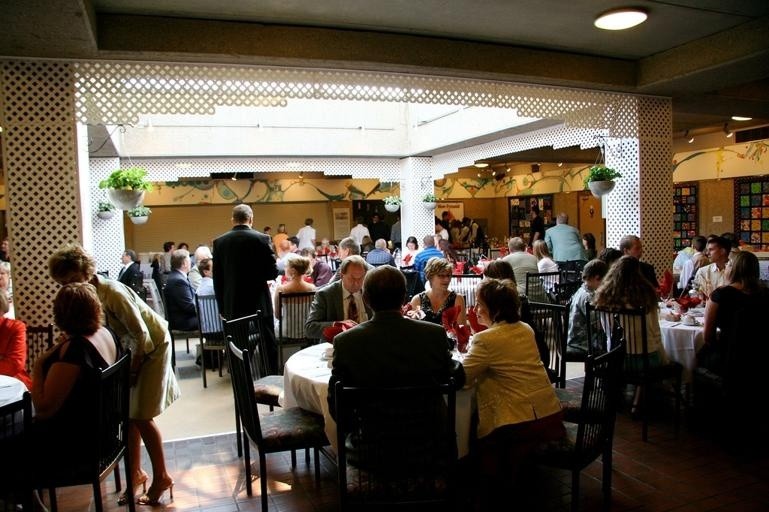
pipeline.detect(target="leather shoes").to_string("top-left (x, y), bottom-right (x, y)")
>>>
top-left (196, 355), bottom-right (202, 365)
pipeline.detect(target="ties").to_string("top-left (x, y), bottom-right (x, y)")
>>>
top-left (118, 267), bottom-right (125, 280)
top-left (348, 295), bottom-right (360, 324)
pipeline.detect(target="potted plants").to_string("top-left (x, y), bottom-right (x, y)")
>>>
top-left (99, 168), bottom-right (153, 210)
top-left (423, 194), bottom-right (437, 210)
top-left (384, 196), bottom-right (402, 213)
top-left (128, 207), bottom-right (153, 226)
top-left (96, 201), bottom-right (117, 220)
top-left (584, 166), bottom-right (623, 195)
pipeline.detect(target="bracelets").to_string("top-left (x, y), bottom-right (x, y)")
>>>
top-left (130, 371), bottom-right (140, 377)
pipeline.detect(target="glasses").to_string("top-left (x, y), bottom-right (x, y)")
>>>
top-left (431, 274), bottom-right (452, 278)
top-left (724, 263), bottom-right (732, 267)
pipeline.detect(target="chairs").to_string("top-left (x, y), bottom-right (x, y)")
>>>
top-left (336, 376), bottom-right (458, 512)
top-left (25, 323), bottom-right (54, 377)
top-left (162, 283), bottom-right (201, 374)
top-left (279, 290), bottom-right (321, 372)
top-left (525, 300), bottom-right (571, 390)
top-left (0, 392), bottom-right (33, 512)
top-left (525, 270), bottom-right (563, 327)
top-left (502, 338), bottom-right (627, 512)
top-left (698, 313), bottom-right (769, 437)
top-left (451, 272), bottom-right (485, 317)
top-left (560, 310), bottom-right (623, 424)
top-left (556, 259), bottom-right (588, 300)
top-left (35, 348), bottom-right (138, 512)
top-left (225, 334), bottom-right (325, 512)
top-left (222, 309), bottom-right (299, 471)
top-left (194, 292), bottom-right (228, 388)
top-left (584, 300), bottom-right (684, 442)
top-left (150, 280), bottom-right (164, 319)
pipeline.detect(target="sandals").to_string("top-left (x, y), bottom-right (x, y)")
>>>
top-left (631, 405), bottom-right (642, 419)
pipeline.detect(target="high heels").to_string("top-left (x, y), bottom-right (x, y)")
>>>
top-left (137, 475), bottom-right (174, 505)
top-left (117, 468), bottom-right (148, 506)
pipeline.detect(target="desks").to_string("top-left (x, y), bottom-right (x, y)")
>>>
top-left (657, 306), bottom-right (705, 421)
top-left (284, 334), bottom-right (479, 491)
top-left (0, 374), bottom-right (31, 426)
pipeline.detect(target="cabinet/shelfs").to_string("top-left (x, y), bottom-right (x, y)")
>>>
top-left (509, 195), bottom-right (554, 247)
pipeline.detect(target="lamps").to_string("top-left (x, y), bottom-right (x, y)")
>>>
top-left (592, 7), bottom-right (648, 31)
top-left (684, 129), bottom-right (694, 143)
top-left (722, 122), bottom-right (733, 138)
top-left (729, 111), bottom-right (752, 122)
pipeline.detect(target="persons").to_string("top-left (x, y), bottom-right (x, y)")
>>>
top-left (213, 204), bottom-right (279, 377)
top-left (305, 254), bottom-right (373, 343)
top-left (0, 282), bottom-right (122, 512)
top-left (704, 251), bottom-right (769, 378)
top-left (391, 215), bottom-right (401, 248)
top-left (117, 249), bottom-right (140, 285)
top-left (720, 231), bottom-right (741, 261)
top-left (301, 247), bottom-right (333, 285)
top-left (534, 239), bottom-right (559, 293)
top-left (328, 238), bottom-right (376, 286)
top-left (594, 257), bottom-right (678, 416)
top-left (296, 218), bottom-right (317, 251)
top-left (680, 235), bottom-right (709, 293)
top-left (707, 234), bottom-right (720, 242)
top-left (276, 240), bottom-right (300, 270)
top-left (545, 212), bottom-right (584, 261)
top-left (48, 242), bottom-right (181, 506)
top-left (1, 262), bottom-right (16, 318)
top-left (348, 217), bottom-right (369, 253)
top-left (482, 260), bottom-right (550, 369)
top-left (263, 226), bottom-right (271, 235)
top-left (412, 235), bottom-right (443, 284)
top-left (189, 245), bottom-right (212, 292)
top-left (567, 259), bottom-right (604, 351)
top-left (1, 287), bottom-right (32, 401)
top-left (460, 278), bottom-right (565, 455)
top-left (529, 206), bottom-right (545, 245)
top-left (276, 252), bottom-right (319, 338)
top-left (163, 242), bottom-right (176, 272)
top-left (692, 238), bottom-right (734, 296)
top-left (582, 233), bottom-right (597, 260)
top-left (401, 236), bottom-right (422, 265)
top-left (316, 237), bottom-right (333, 257)
top-left (2, 238), bottom-right (10, 262)
top-left (361, 236), bottom-right (373, 260)
top-left (164, 249), bottom-right (220, 368)
top-left (366, 239), bottom-right (397, 268)
top-left (177, 243), bottom-right (189, 249)
top-left (369, 215), bottom-right (384, 239)
top-left (598, 248), bottom-right (622, 269)
top-left (410, 258), bottom-right (464, 326)
top-left (273, 223), bottom-right (288, 254)
top-left (502, 237), bottom-right (549, 302)
top-left (673, 242), bottom-right (695, 270)
top-left (288, 237), bottom-right (301, 254)
top-left (193, 258), bottom-right (221, 333)
top-left (435, 211), bottom-right (485, 262)
top-left (620, 235), bottom-right (643, 259)
top-left (328, 264), bottom-right (465, 474)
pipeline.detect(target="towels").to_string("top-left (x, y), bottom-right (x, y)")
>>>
top-left (404, 254), bottom-right (412, 265)
top-left (677, 297), bottom-right (702, 313)
top-left (469, 266), bottom-right (481, 274)
top-left (455, 262), bottom-right (464, 275)
top-left (331, 251), bottom-right (336, 257)
top-left (304, 276), bottom-right (312, 283)
top-left (442, 304), bottom-right (462, 336)
top-left (323, 320), bottom-right (354, 345)
top-left (527, 247), bottom-right (533, 254)
top-left (456, 324), bottom-right (470, 352)
top-left (469, 306), bottom-right (488, 332)
top-left (325, 247), bottom-right (330, 255)
top-left (282, 275), bottom-right (286, 284)
top-left (658, 270), bottom-right (673, 299)
top-left (500, 247), bottom-right (508, 257)
